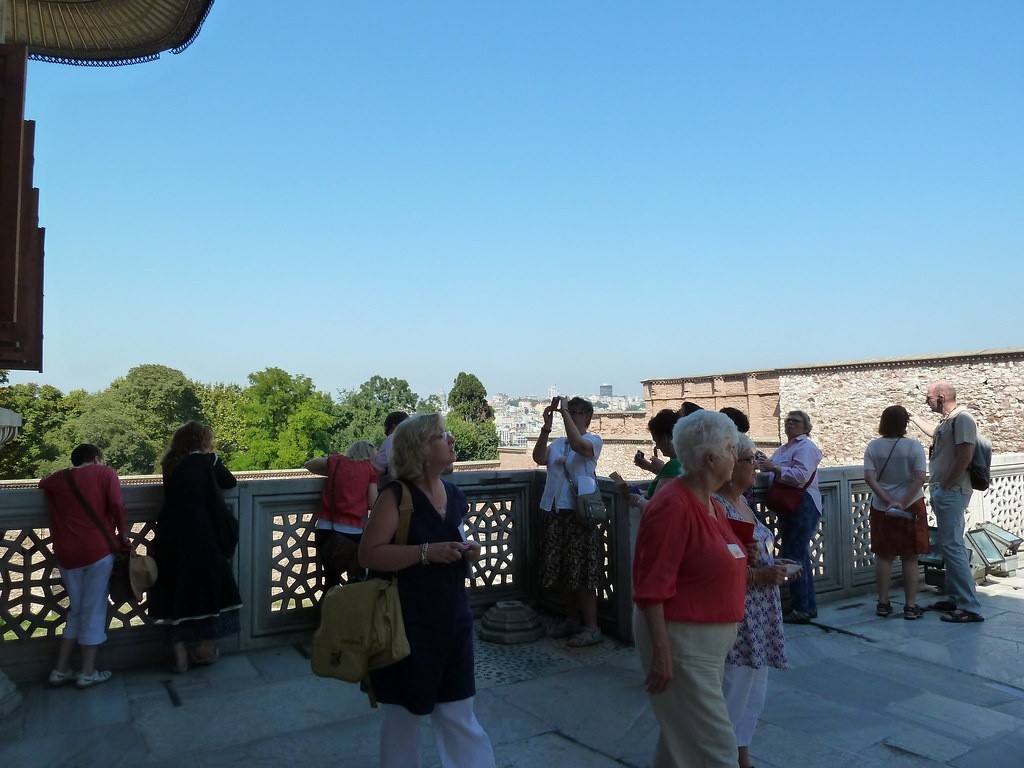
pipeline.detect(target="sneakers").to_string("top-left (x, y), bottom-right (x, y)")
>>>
top-left (783, 610), bottom-right (811, 625)
top-left (49, 667), bottom-right (83, 687)
top-left (812, 610), bottom-right (818, 618)
top-left (76, 669), bottom-right (111, 688)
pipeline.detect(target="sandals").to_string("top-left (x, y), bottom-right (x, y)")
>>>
top-left (940, 610), bottom-right (985, 622)
top-left (549, 615), bottom-right (585, 637)
top-left (903, 603), bottom-right (922, 619)
top-left (928, 601), bottom-right (957, 611)
top-left (567, 627), bottom-right (604, 646)
top-left (877, 601), bottom-right (893, 615)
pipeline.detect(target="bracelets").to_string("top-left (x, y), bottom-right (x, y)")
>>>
top-left (541, 427), bottom-right (551, 433)
top-left (421, 543), bottom-right (429, 566)
top-left (637, 498), bottom-right (644, 507)
top-left (562, 409), bottom-right (569, 417)
top-left (773, 465), bottom-right (777, 473)
top-left (748, 565), bottom-right (755, 585)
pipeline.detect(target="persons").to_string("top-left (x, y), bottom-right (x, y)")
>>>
top-left (306, 441), bottom-right (379, 593)
top-left (375, 411), bottom-right (453, 475)
top-left (533, 395), bottom-right (603, 646)
top-left (908, 381), bottom-right (985, 622)
top-left (758, 411), bottom-right (822, 622)
top-left (719, 407), bottom-right (757, 517)
top-left (629, 401), bottom-right (702, 512)
top-left (710, 434), bottom-right (804, 768)
top-left (148, 421), bottom-right (243, 672)
top-left (632, 410), bottom-right (759, 768)
top-left (358, 413), bottom-right (494, 768)
top-left (863, 406), bottom-right (930, 620)
top-left (37, 444), bottom-right (136, 688)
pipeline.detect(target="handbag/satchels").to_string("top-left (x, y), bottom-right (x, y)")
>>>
top-left (320, 534), bottom-right (361, 570)
top-left (108, 552), bottom-right (133, 605)
top-left (576, 492), bottom-right (607, 526)
top-left (311, 579), bottom-right (411, 683)
top-left (766, 482), bottom-right (803, 513)
top-left (212, 507), bottom-right (238, 558)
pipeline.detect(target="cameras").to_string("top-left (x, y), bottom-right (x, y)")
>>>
top-left (456, 542), bottom-right (474, 551)
top-left (634, 449), bottom-right (645, 465)
top-left (754, 451), bottom-right (767, 460)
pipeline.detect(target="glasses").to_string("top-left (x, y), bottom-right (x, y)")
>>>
top-left (738, 458), bottom-right (756, 464)
top-left (428, 429), bottom-right (452, 443)
top-left (569, 411), bottom-right (585, 416)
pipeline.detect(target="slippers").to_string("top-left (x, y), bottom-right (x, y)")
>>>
top-left (170, 656), bottom-right (188, 673)
top-left (188, 649), bottom-right (219, 665)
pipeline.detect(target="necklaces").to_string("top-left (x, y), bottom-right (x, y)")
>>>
top-left (433, 480), bottom-right (446, 513)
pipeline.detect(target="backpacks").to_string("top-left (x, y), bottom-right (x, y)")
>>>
top-left (952, 410), bottom-right (991, 491)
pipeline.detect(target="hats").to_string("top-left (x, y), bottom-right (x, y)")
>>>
top-left (130, 551), bottom-right (158, 602)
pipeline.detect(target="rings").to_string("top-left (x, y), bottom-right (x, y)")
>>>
top-left (762, 461), bottom-right (763, 463)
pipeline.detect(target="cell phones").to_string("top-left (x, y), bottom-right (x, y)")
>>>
top-left (550, 397), bottom-right (560, 411)
